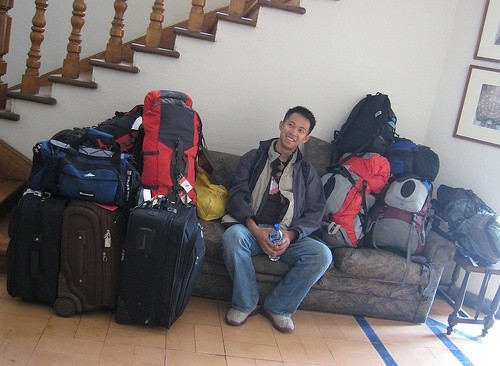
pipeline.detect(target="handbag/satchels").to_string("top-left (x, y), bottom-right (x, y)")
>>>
top-left (195, 166), bottom-right (229, 221)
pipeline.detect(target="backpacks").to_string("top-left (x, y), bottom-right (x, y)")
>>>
top-left (387, 136), bottom-right (440, 182)
top-left (366, 176), bottom-right (436, 304)
top-left (331, 92), bottom-right (400, 160)
top-left (320, 153), bottom-right (391, 249)
top-left (27, 90), bottom-right (208, 206)
top-left (425, 184), bottom-right (500, 267)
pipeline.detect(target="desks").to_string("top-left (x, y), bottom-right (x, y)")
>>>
top-left (431, 225), bottom-right (500, 338)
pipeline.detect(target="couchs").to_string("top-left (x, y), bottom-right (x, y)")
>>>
top-left (190, 151), bottom-right (456, 322)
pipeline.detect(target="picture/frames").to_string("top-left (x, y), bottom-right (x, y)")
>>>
top-left (453, 0), bottom-right (500, 148)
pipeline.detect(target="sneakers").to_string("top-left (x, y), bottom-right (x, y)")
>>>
top-left (225, 305), bottom-right (257, 326)
top-left (258, 303), bottom-right (294, 332)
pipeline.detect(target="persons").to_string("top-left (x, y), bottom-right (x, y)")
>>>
top-left (221, 107), bottom-right (332, 331)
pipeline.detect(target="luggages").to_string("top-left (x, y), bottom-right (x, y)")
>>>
top-left (6, 189), bottom-right (67, 303)
top-left (117, 197), bottom-right (206, 328)
top-left (54, 201), bottom-right (125, 318)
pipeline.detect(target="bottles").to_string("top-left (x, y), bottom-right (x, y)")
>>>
top-left (387, 117), bottom-right (396, 130)
top-left (269, 224), bottom-right (283, 262)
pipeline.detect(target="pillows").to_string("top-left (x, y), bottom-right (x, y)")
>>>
top-left (303, 136), bottom-right (338, 178)
